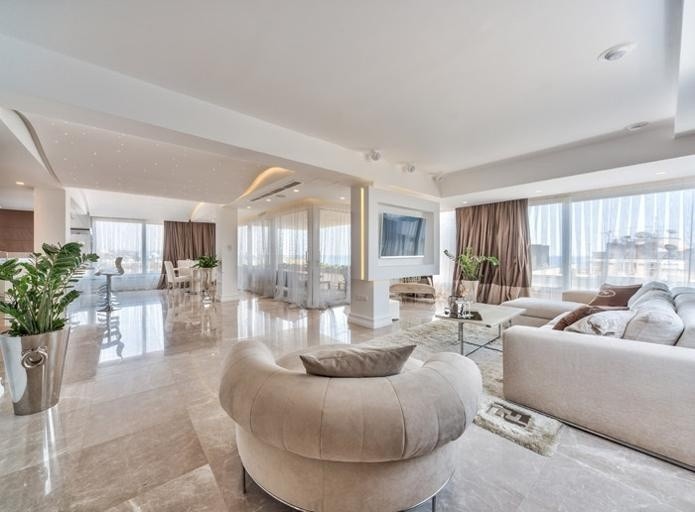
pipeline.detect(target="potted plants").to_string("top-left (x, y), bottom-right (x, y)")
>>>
top-left (443, 246), bottom-right (500, 304)
top-left (0, 239), bottom-right (101, 417)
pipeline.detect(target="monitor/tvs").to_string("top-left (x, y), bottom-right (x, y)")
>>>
top-left (380, 212), bottom-right (427, 258)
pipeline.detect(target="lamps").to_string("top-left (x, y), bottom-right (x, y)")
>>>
top-left (599, 44), bottom-right (631, 67)
top-left (628, 121), bottom-right (648, 134)
top-left (368, 149), bottom-right (444, 185)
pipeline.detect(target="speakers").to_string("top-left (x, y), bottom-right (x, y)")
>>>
top-left (409, 164), bottom-right (416, 176)
top-left (369, 150), bottom-right (383, 163)
top-left (436, 176), bottom-right (443, 183)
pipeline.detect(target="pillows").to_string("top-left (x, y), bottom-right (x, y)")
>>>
top-left (622, 289), bottom-right (685, 345)
top-left (589, 282), bottom-right (643, 309)
top-left (299, 339), bottom-right (418, 377)
top-left (564, 310), bottom-right (638, 339)
top-left (553, 304), bottom-right (604, 332)
top-left (626, 279), bottom-right (669, 308)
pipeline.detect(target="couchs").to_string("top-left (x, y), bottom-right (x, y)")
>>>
top-left (501, 286), bottom-right (695, 471)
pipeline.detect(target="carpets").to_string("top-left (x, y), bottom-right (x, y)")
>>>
top-left (363, 318), bottom-right (566, 457)
top-left (473, 397), bottom-right (563, 457)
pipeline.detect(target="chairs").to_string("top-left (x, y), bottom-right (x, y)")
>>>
top-left (220, 339), bottom-right (483, 512)
top-left (164, 256), bottom-right (219, 306)
top-left (95, 256), bottom-right (124, 311)
